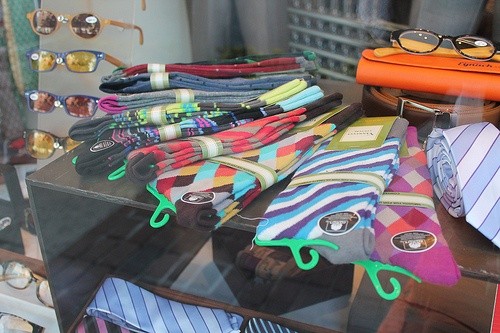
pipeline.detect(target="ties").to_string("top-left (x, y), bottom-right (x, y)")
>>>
top-left (425, 122), bottom-right (500, 249)
top-left (74, 316), bottom-right (139, 333)
top-left (244, 318), bottom-right (297, 332)
top-left (85, 278), bottom-right (245, 333)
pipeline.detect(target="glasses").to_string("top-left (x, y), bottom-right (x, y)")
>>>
top-left (26, 50), bottom-right (129, 73)
top-left (0, 260), bottom-right (54, 308)
top-left (21, 129), bottom-right (84, 160)
top-left (24, 90), bottom-right (102, 119)
top-left (374, 27), bottom-right (500, 63)
top-left (0, 311), bottom-right (46, 332)
top-left (26, 9), bottom-right (145, 45)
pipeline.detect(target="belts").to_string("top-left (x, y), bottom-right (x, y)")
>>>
top-left (361, 85), bottom-right (500, 131)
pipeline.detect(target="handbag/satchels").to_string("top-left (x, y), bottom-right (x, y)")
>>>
top-left (345, 259), bottom-right (499, 333)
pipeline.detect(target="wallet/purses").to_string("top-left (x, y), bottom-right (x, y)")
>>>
top-left (356, 48), bottom-right (500, 101)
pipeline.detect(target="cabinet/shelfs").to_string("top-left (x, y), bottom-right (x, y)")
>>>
top-left (26, 80), bottom-right (499, 333)
